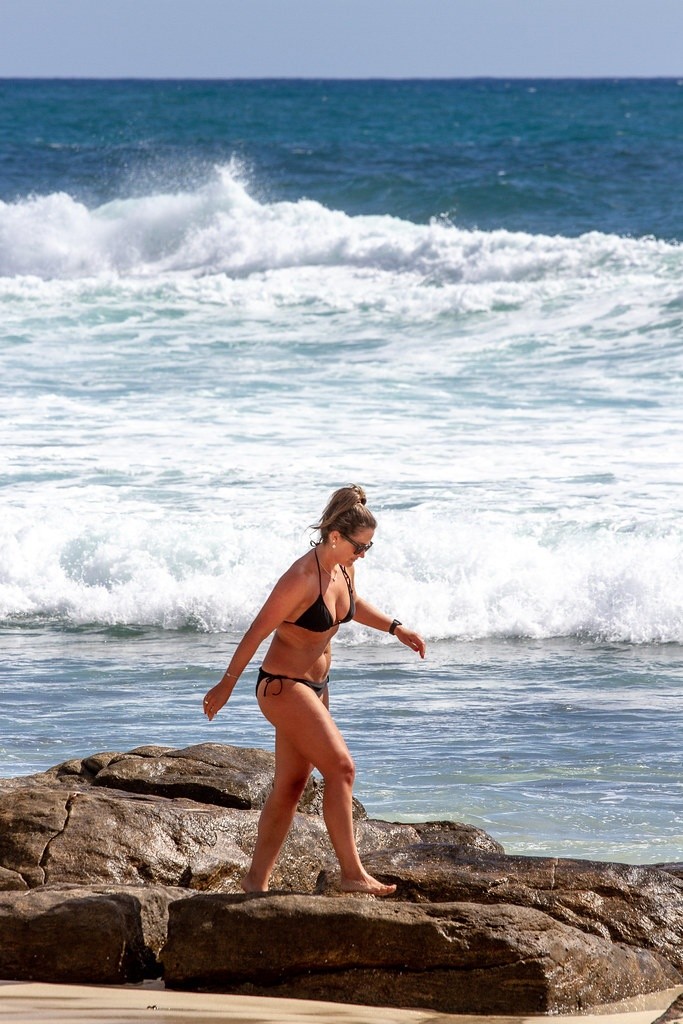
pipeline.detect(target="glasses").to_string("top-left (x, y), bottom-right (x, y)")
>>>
top-left (339, 532), bottom-right (373, 555)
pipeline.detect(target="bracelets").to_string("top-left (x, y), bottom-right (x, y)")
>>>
top-left (223, 673), bottom-right (239, 680)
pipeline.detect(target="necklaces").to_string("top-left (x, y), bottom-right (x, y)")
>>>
top-left (319, 561), bottom-right (337, 581)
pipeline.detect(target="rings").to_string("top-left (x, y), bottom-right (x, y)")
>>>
top-left (421, 639), bottom-right (425, 642)
top-left (204, 701), bottom-right (208, 704)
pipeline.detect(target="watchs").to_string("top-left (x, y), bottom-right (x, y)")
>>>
top-left (390, 619), bottom-right (402, 636)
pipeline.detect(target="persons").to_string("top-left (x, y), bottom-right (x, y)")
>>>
top-left (203, 485), bottom-right (426, 894)
